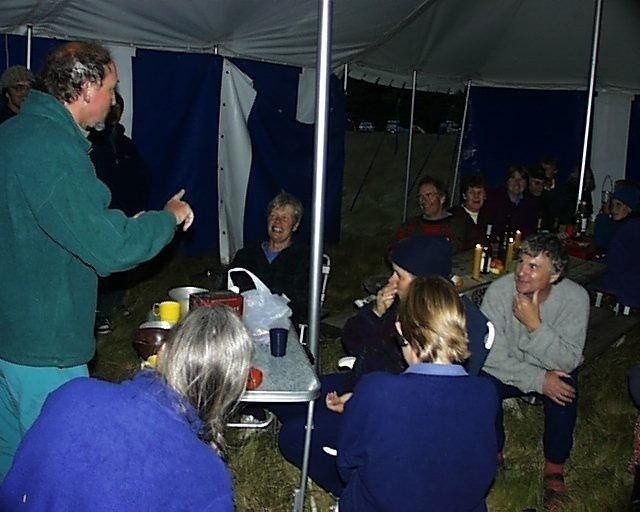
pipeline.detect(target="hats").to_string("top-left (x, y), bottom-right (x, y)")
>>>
top-left (388, 234), bottom-right (456, 285)
top-left (611, 187), bottom-right (636, 212)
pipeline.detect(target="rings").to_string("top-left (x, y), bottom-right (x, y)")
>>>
top-left (380, 293), bottom-right (383, 298)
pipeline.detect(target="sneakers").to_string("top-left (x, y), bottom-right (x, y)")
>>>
top-left (538, 465), bottom-right (571, 511)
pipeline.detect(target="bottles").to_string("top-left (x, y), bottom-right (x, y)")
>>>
top-left (579, 201), bottom-right (587, 233)
top-left (502, 215), bottom-right (511, 247)
top-left (479, 225), bottom-right (493, 273)
top-left (574, 210), bottom-right (582, 239)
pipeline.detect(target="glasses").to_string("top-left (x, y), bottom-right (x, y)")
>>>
top-left (415, 191), bottom-right (440, 203)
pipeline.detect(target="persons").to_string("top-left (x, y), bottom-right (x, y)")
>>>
top-left (488, 165), bottom-right (540, 236)
top-left (445, 180), bottom-right (490, 257)
top-left (587, 185), bottom-right (640, 308)
top-left (544, 153), bottom-right (590, 231)
top-left (1, 65), bottom-right (35, 123)
top-left (1, 302), bottom-right (252, 512)
top-left (527, 171), bottom-right (551, 230)
top-left (269, 236), bottom-right (457, 497)
top-left (478, 232), bottom-right (591, 512)
top-left (83, 93), bottom-right (153, 340)
top-left (325, 276), bottom-right (501, 512)
top-left (386, 174), bottom-right (465, 266)
top-left (0, 39), bottom-right (195, 479)
top-left (220, 192), bottom-right (311, 422)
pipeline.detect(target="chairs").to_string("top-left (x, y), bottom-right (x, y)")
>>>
top-left (586, 282), bottom-right (630, 318)
top-left (294, 253), bottom-right (331, 346)
top-left (452, 293), bottom-right (495, 377)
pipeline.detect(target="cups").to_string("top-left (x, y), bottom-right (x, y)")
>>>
top-left (152, 301), bottom-right (180, 325)
top-left (269, 328), bottom-right (289, 357)
